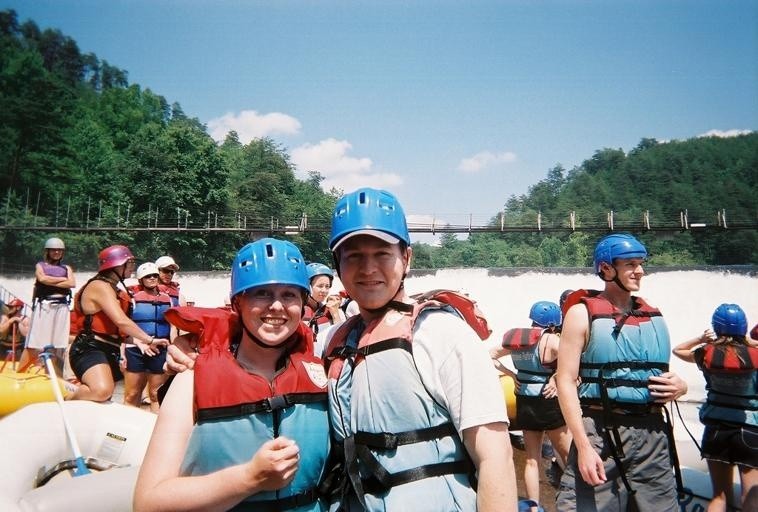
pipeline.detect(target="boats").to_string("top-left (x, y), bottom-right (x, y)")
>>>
top-left (0, 399), bottom-right (156, 512)
top-left (673, 462), bottom-right (746, 512)
top-left (499, 375), bottom-right (522, 424)
top-left (0, 360), bottom-right (78, 418)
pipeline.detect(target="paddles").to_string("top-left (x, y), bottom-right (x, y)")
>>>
top-left (37, 352), bottom-right (91, 477)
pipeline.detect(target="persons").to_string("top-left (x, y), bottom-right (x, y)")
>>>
top-left (558, 234), bottom-right (687, 511)
top-left (671, 304), bottom-right (758, 512)
top-left (560, 289), bottom-right (575, 309)
top-left (16, 237), bottom-right (192, 415)
top-left (164, 187), bottom-right (519, 512)
top-left (1, 298), bottom-right (31, 362)
top-left (489, 300), bottom-right (575, 511)
top-left (296, 257), bottom-right (364, 363)
top-left (134, 238), bottom-right (331, 512)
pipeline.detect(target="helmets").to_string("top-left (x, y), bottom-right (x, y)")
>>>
top-left (530, 301), bottom-right (561, 327)
top-left (230, 187), bottom-right (410, 299)
top-left (6, 238), bottom-right (179, 309)
top-left (593, 234), bottom-right (646, 272)
top-left (712, 303), bottom-right (748, 336)
